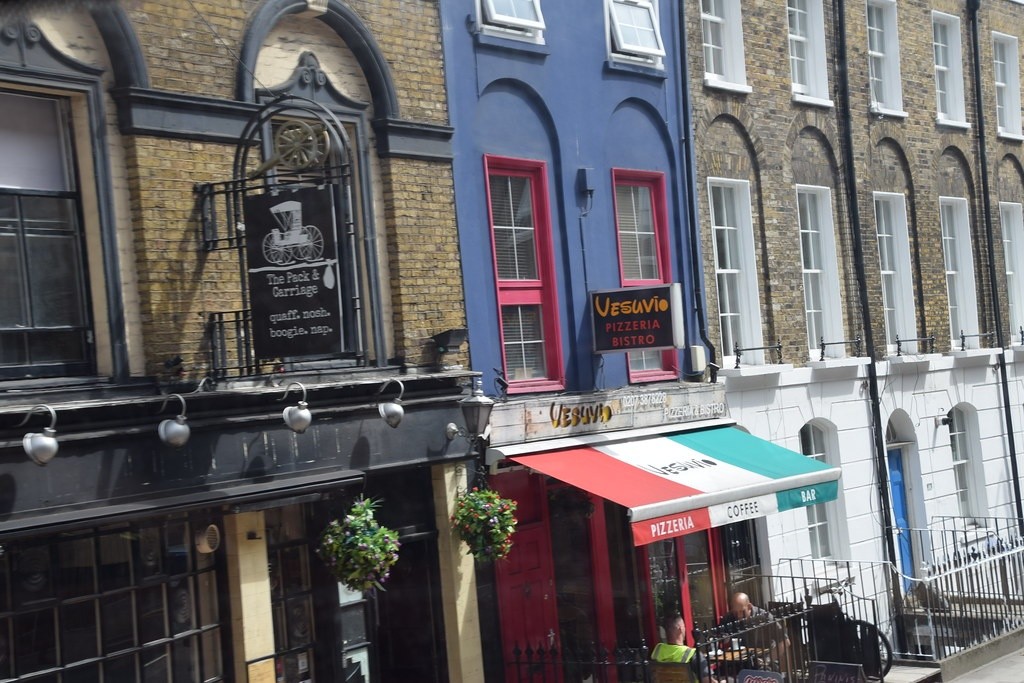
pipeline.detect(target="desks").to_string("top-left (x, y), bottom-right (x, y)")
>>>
top-left (703, 648), bottom-right (772, 669)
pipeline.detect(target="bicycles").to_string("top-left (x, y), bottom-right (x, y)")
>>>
top-left (818, 575), bottom-right (893, 679)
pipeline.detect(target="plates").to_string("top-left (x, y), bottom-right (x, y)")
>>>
top-left (709, 651), bottom-right (723, 656)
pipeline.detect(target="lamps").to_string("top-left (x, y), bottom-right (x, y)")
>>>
top-left (11, 404), bottom-right (60, 466)
top-left (446, 374), bottom-right (494, 444)
top-left (277, 382), bottom-right (312, 434)
top-left (155, 393), bottom-right (190, 449)
top-left (372, 379), bottom-right (404, 429)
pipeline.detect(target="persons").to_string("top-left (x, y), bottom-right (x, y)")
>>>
top-left (647, 613), bottom-right (736, 683)
top-left (714, 593), bottom-right (791, 676)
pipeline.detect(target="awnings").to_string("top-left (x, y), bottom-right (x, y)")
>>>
top-left (507, 427), bottom-right (842, 547)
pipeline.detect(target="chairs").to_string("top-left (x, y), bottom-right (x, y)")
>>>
top-left (768, 601), bottom-right (801, 671)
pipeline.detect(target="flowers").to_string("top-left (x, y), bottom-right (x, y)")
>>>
top-left (314, 496), bottom-right (400, 594)
top-left (451, 487), bottom-right (519, 563)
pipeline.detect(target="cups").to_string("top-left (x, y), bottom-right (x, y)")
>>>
top-left (732, 638), bottom-right (741, 650)
